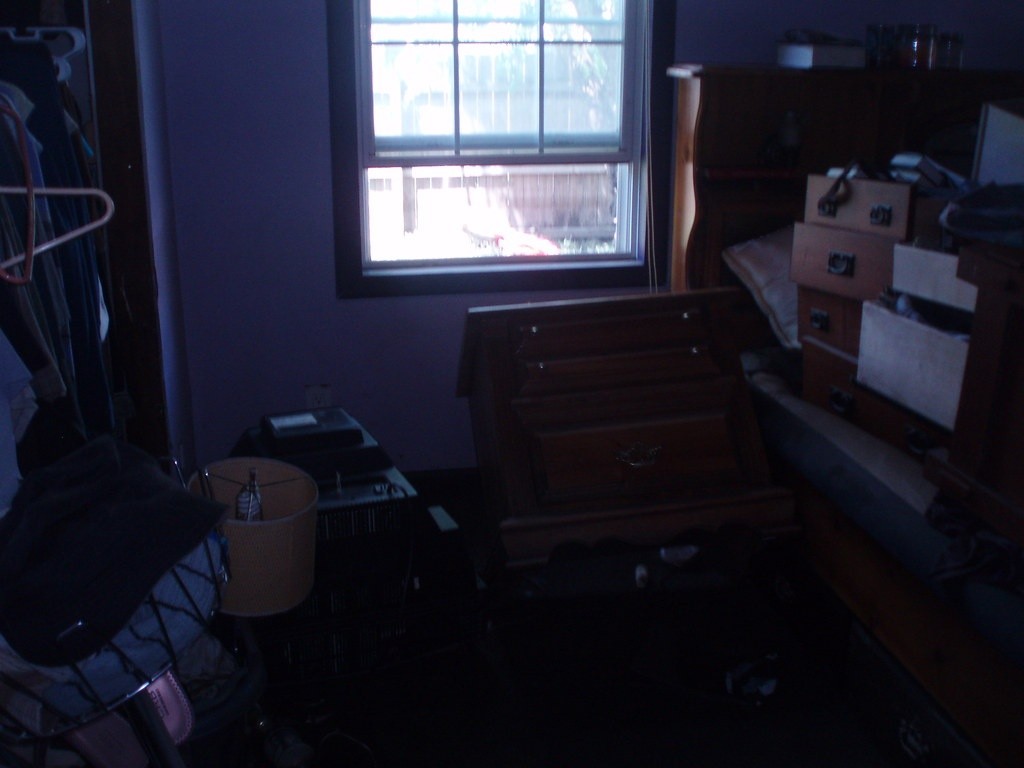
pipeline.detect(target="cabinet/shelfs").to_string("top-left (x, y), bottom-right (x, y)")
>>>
top-left (665, 64), bottom-right (1024, 292)
top-left (453, 294), bottom-right (797, 570)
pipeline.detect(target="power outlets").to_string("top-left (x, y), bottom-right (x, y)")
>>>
top-left (304, 382), bottom-right (333, 412)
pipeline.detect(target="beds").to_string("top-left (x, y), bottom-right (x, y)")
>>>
top-left (743, 370), bottom-right (1024, 768)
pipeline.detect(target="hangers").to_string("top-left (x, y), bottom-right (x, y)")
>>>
top-left (0, 102), bottom-right (115, 285)
top-left (0, 24), bottom-right (87, 84)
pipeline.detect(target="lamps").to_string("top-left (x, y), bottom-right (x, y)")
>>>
top-left (201, 455), bottom-right (318, 619)
